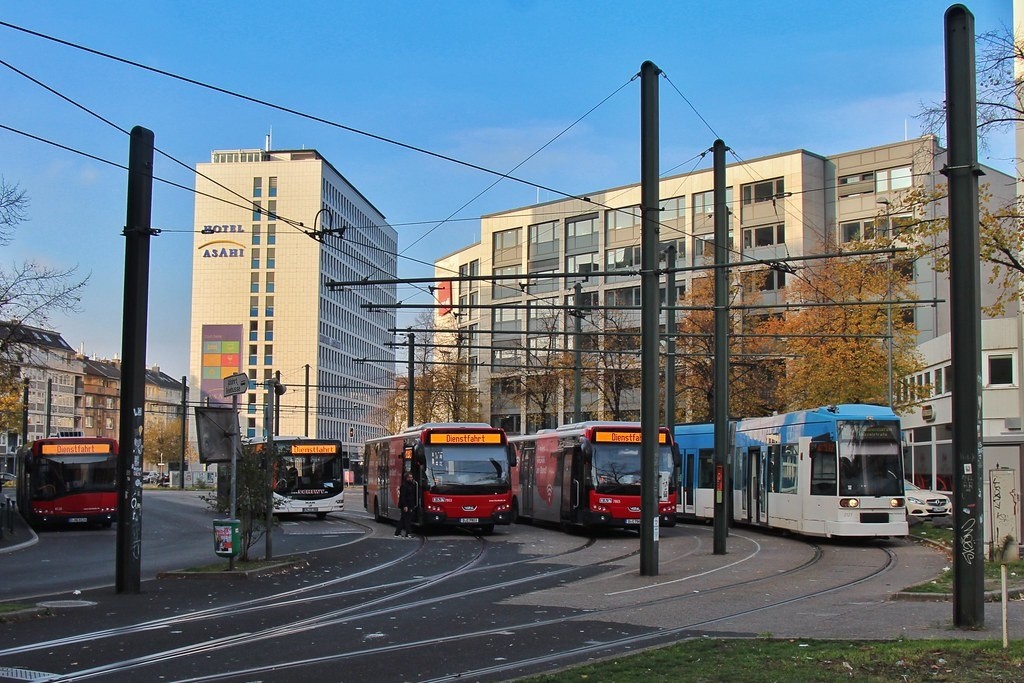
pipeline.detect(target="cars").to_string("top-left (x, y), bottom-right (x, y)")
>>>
top-left (904, 478), bottom-right (953, 516)
top-left (143, 471), bottom-right (157, 483)
top-left (161, 476), bottom-right (170, 486)
top-left (0, 472), bottom-right (16, 483)
top-left (156, 475), bottom-right (165, 483)
top-left (157, 473), bottom-right (163, 476)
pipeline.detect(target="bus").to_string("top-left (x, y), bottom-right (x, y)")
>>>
top-left (362, 423), bottom-right (517, 534)
top-left (16, 436), bottom-right (119, 529)
top-left (218, 436), bottom-right (350, 520)
top-left (508, 422), bottom-right (681, 533)
top-left (675, 403), bottom-right (909, 539)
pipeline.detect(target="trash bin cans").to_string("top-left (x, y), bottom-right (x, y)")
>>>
top-left (213, 518), bottom-right (241, 559)
top-left (343, 468), bottom-right (355, 484)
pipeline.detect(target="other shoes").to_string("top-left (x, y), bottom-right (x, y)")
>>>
top-left (394, 534), bottom-right (404, 538)
top-left (406, 534), bottom-right (416, 540)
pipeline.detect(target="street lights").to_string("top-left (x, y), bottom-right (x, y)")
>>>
top-left (876, 197), bottom-right (893, 409)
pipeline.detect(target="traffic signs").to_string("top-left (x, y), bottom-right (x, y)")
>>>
top-left (223, 372), bottom-right (250, 397)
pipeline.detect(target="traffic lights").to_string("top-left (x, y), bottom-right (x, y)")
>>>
top-left (349, 427), bottom-right (354, 438)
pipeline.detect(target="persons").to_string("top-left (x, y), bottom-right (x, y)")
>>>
top-left (394, 472), bottom-right (417, 539)
top-left (315, 470), bottom-right (325, 484)
